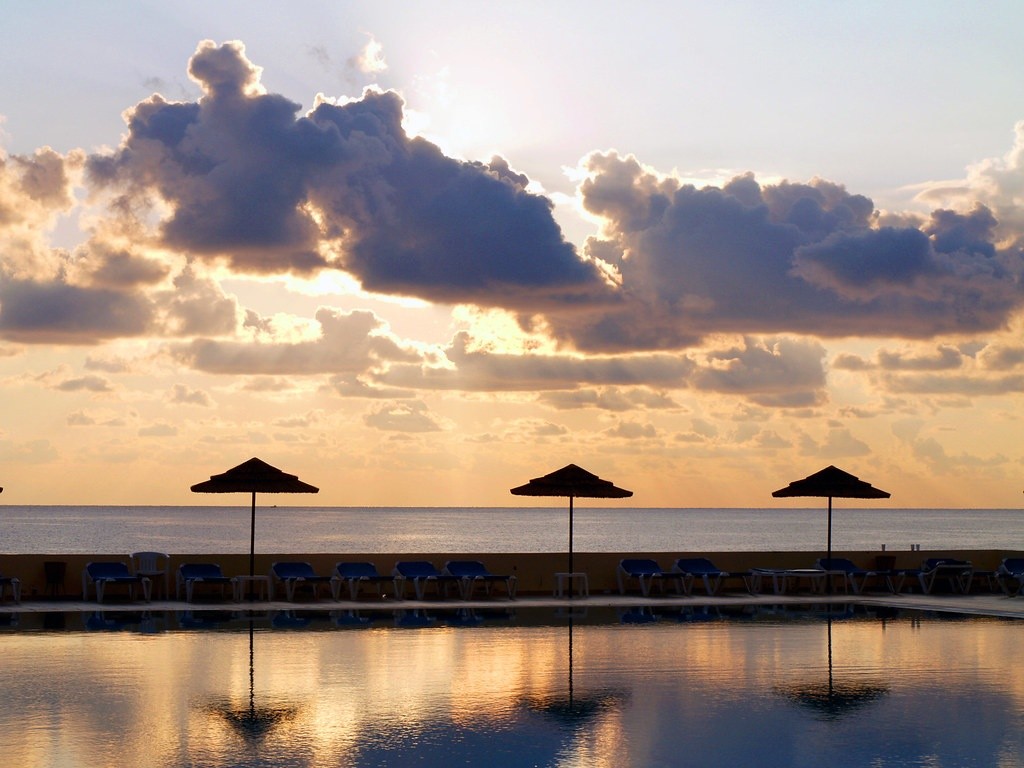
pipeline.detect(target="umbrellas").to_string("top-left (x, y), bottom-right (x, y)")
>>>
top-left (189, 457), bottom-right (320, 601)
top-left (510, 463), bottom-right (634, 599)
top-left (770, 465), bottom-right (891, 570)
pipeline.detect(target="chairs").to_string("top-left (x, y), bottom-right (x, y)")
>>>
top-left (817, 558), bottom-right (899, 596)
top-left (83, 561), bottom-right (140, 604)
top-left (129, 552), bottom-right (170, 601)
top-left (875, 555), bottom-right (968, 597)
top-left (272, 562), bottom-right (342, 602)
top-left (615, 559), bottom-right (688, 598)
top-left (674, 558), bottom-right (753, 598)
top-left (995, 558), bottom-right (1024, 598)
top-left (748, 569), bottom-right (828, 594)
top-left (0, 575), bottom-right (24, 605)
top-left (442, 563), bottom-right (517, 600)
top-left (333, 561), bottom-right (406, 600)
top-left (175, 562), bottom-right (238, 602)
top-left (392, 561), bottom-right (471, 600)
top-left (930, 558), bottom-right (994, 595)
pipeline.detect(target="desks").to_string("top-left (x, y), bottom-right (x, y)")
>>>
top-left (828, 567), bottom-right (847, 594)
top-left (553, 573), bottom-right (589, 598)
top-left (42, 561), bottom-right (68, 601)
top-left (234, 574), bottom-right (275, 602)
